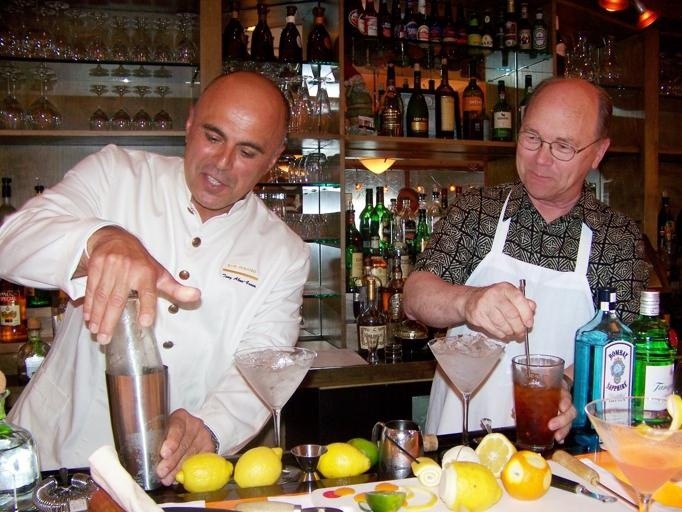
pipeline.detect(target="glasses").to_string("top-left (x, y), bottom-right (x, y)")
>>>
top-left (516, 131), bottom-right (605, 162)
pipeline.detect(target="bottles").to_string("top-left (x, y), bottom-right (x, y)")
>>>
top-left (0, 177), bottom-right (51, 384)
top-left (222, 2), bottom-right (333, 62)
top-left (0, 369), bottom-right (42, 502)
top-left (555, 11), bottom-right (624, 85)
top-left (570, 287), bottom-right (676, 453)
top-left (660, 63), bottom-right (682, 96)
top-left (345, 185), bottom-right (463, 363)
top-left (344, 0), bottom-right (547, 141)
top-left (659, 190), bottom-right (682, 281)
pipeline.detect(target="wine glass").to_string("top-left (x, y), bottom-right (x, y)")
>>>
top-left (428, 335), bottom-right (508, 460)
top-left (585, 396), bottom-right (682, 512)
top-left (1, 1), bottom-right (198, 130)
top-left (233, 346), bottom-right (317, 486)
top-left (291, 444), bottom-right (328, 482)
top-left (224, 64), bottom-right (332, 135)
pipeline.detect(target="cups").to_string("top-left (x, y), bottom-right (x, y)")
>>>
top-left (372, 420), bottom-right (422, 480)
top-left (512, 355), bottom-right (565, 454)
top-left (105, 297), bottom-right (168, 490)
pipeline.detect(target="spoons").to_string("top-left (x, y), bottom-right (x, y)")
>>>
top-left (520, 279), bottom-right (547, 389)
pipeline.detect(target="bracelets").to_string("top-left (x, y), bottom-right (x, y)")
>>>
top-left (204, 425), bottom-right (219, 453)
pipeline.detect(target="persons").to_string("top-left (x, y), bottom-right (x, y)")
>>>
top-left (401, 72), bottom-right (648, 435)
top-left (0, 69), bottom-right (311, 487)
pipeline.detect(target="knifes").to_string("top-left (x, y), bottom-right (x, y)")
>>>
top-left (552, 474), bottom-right (617, 503)
top-left (234, 500), bottom-right (344, 512)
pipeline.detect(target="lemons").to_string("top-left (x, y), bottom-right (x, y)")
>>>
top-left (476, 433), bottom-right (518, 479)
top-left (635, 395), bottom-right (682, 441)
top-left (349, 436), bottom-right (378, 468)
top-left (316, 443), bottom-right (370, 482)
top-left (232, 446), bottom-right (282, 489)
top-left (437, 461), bottom-right (504, 512)
top-left (176, 451), bottom-right (234, 493)
top-left (365, 491), bottom-right (405, 512)
